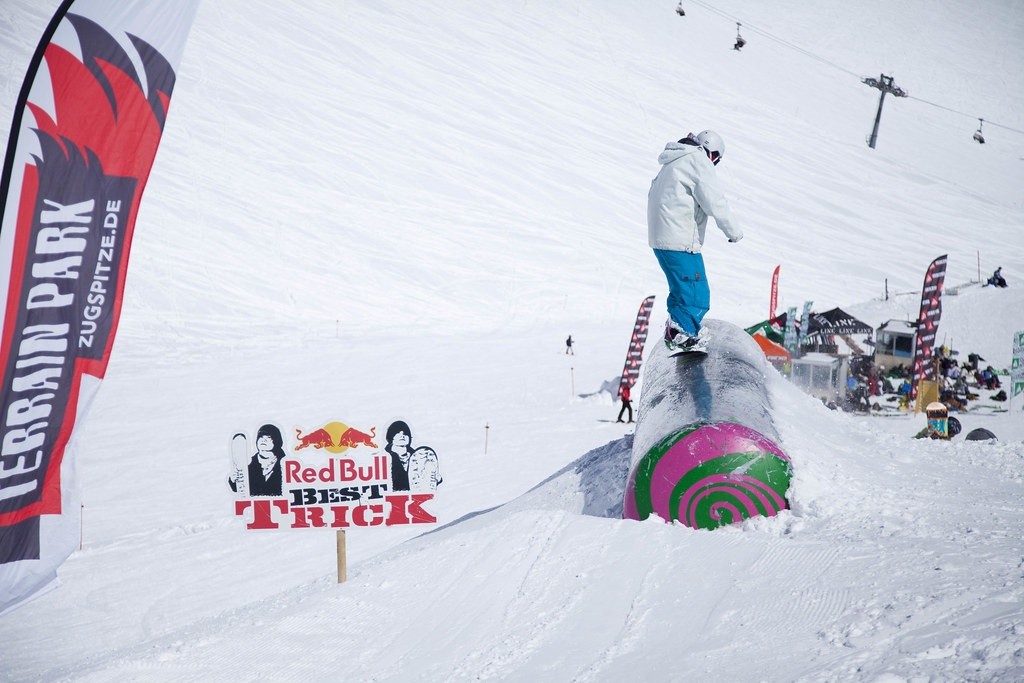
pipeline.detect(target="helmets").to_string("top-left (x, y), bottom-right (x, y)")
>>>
top-left (698, 129), bottom-right (725, 165)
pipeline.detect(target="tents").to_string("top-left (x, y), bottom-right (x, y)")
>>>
top-left (739, 307), bottom-right (872, 357)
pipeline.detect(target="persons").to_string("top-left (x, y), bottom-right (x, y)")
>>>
top-left (617, 382), bottom-right (636, 422)
top-left (994, 266), bottom-right (1009, 288)
top-left (566, 335), bottom-right (575, 355)
top-left (869, 365), bottom-right (885, 394)
top-left (648, 129), bottom-right (744, 351)
top-left (948, 359), bottom-right (961, 376)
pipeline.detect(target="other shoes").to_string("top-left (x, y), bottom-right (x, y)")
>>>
top-left (628, 420), bottom-right (634, 424)
top-left (664, 323), bottom-right (709, 351)
top-left (617, 419), bottom-right (624, 423)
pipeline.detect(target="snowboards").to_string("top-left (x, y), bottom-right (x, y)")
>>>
top-left (667, 340), bottom-right (708, 359)
